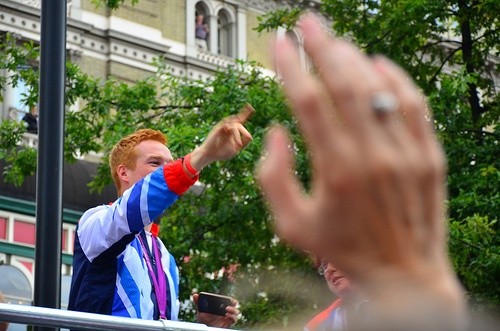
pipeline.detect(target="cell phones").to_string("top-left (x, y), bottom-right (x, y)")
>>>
top-left (198, 292), bottom-right (234, 316)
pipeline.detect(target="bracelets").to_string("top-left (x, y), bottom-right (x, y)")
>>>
top-left (184, 154), bottom-right (198, 175)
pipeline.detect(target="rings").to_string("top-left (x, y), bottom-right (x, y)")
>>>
top-left (370, 94), bottom-right (401, 112)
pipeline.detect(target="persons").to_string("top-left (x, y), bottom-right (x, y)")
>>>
top-left (67, 104), bottom-right (255, 331)
top-left (19, 105), bottom-right (39, 135)
top-left (301, 255), bottom-right (356, 331)
top-left (255, 10), bottom-right (497, 331)
top-left (196, 14), bottom-right (210, 40)
